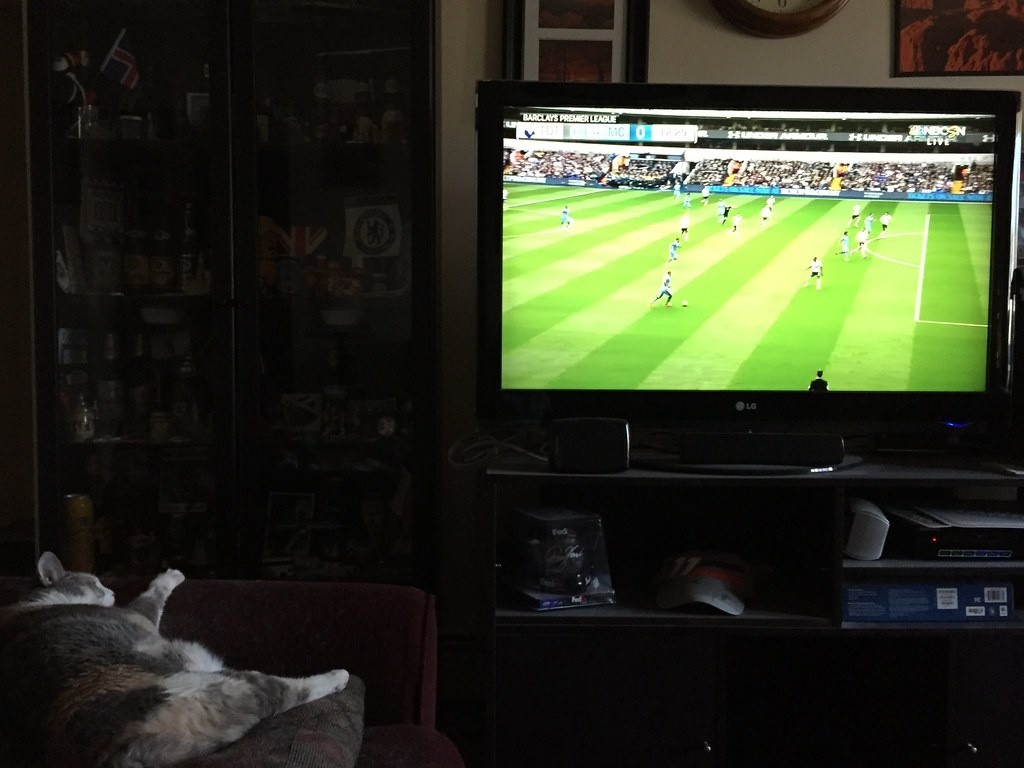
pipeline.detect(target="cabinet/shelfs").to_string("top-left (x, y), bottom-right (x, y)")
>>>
top-left (473, 442), bottom-right (1024, 768)
top-left (28, 0), bottom-right (441, 588)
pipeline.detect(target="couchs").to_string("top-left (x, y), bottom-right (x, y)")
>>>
top-left (0, 575), bottom-right (468, 768)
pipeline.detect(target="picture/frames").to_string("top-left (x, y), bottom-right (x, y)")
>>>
top-left (501, 0), bottom-right (650, 83)
top-left (894, 0), bottom-right (1024, 78)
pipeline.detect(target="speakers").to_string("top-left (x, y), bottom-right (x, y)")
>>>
top-left (842, 497), bottom-right (889, 559)
top-left (548, 417), bottom-right (630, 473)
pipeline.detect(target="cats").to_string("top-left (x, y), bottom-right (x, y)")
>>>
top-left (1, 551), bottom-right (350, 768)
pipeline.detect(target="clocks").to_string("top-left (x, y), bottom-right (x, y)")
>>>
top-left (710, 0), bottom-right (849, 40)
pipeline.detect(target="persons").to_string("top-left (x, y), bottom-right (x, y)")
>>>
top-left (807, 370), bottom-right (829, 392)
top-left (502, 148), bottom-right (993, 290)
top-left (651, 272), bottom-right (673, 307)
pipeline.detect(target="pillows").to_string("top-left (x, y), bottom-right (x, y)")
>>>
top-left (176, 675), bottom-right (367, 768)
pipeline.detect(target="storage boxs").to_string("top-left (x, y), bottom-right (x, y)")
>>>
top-left (831, 575), bottom-right (1016, 622)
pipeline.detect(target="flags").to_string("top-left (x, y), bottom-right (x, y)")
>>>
top-left (100, 29), bottom-right (141, 93)
top-left (257, 217), bottom-right (365, 297)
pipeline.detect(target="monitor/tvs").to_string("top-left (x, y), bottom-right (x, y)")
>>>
top-left (474, 78), bottom-right (1024, 476)
